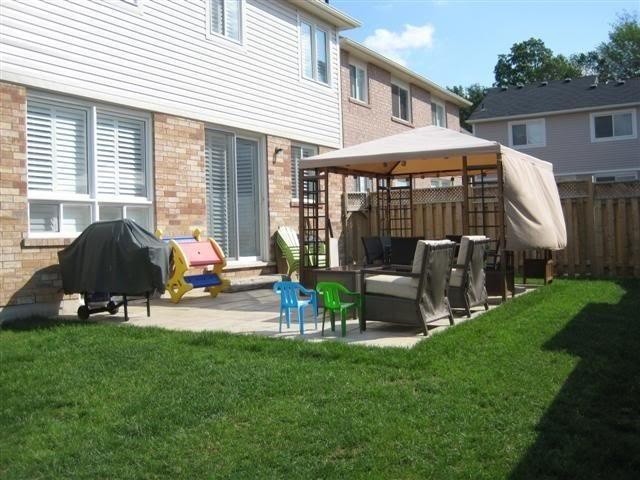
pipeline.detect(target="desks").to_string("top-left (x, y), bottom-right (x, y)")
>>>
top-left (310, 264), bottom-right (391, 320)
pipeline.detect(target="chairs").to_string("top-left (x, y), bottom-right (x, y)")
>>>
top-left (272, 280), bottom-right (365, 337)
top-left (276, 226), bottom-right (326, 277)
top-left (361, 233), bottom-right (491, 336)
